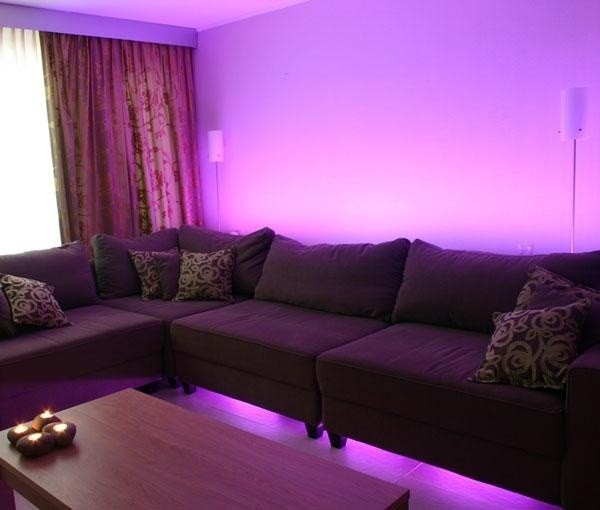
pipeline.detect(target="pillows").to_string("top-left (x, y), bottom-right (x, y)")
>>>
top-left (124, 245), bottom-right (238, 304)
top-left (465, 262), bottom-right (600, 394)
top-left (0, 272), bottom-right (76, 338)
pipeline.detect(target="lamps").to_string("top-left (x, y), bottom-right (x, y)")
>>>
top-left (206, 130), bottom-right (226, 232)
top-left (7, 407), bottom-right (77, 459)
top-left (560, 85), bottom-right (588, 252)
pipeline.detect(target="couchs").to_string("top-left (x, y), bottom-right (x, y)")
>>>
top-left (0, 223), bottom-right (600, 509)
